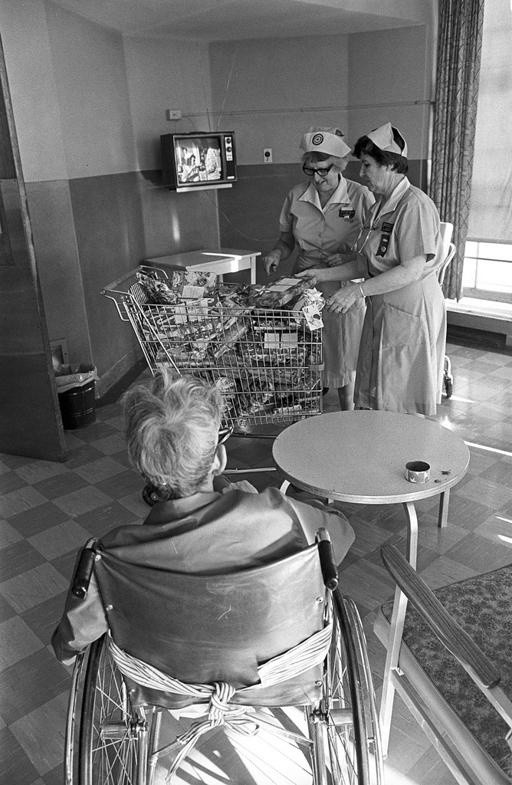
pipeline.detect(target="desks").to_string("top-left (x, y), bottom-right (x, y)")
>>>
top-left (273, 410), bottom-right (471, 570)
top-left (144, 248), bottom-right (262, 286)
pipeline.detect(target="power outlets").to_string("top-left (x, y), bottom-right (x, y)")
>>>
top-left (168, 110), bottom-right (182, 120)
top-left (264, 148), bottom-right (272, 163)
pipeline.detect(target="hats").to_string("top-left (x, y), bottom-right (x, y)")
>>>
top-left (299, 126), bottom-right (351, 159)
top-left (366, 122), bottom-right (408, 159)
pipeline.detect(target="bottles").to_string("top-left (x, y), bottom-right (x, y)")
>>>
top-left (208, 316), bottom-right (253, 358)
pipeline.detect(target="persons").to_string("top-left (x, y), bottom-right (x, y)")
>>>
top-left (52, 367), bottom-right (356, 671)
top-left (175, 139), bottom-right (203, 183)
top-left (263, 131), bottom-right (377, 410)
top-left (295, 121), bottom-right (449, 419)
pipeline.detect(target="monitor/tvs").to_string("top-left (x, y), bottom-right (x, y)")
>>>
top-left (158, 129), bottom-right (237, 188)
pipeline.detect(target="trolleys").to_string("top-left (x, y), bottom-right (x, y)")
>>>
top-left (100, 263), bottom-right (324, 505)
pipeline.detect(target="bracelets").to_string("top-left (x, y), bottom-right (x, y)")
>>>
top-left (358, 283), bottom-right (367, 300)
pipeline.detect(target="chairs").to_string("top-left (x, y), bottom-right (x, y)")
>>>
top-left (370, 541), bottom-right (512, 785)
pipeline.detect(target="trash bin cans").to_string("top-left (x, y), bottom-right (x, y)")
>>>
top-left (54, 362), bottom-right (101, 430)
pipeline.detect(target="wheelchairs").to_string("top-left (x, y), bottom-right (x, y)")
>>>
top-left (53, 522), bottom-right (387, 784)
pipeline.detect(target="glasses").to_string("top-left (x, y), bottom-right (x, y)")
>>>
top-left (302, 161), bottom-right (334, 177)
